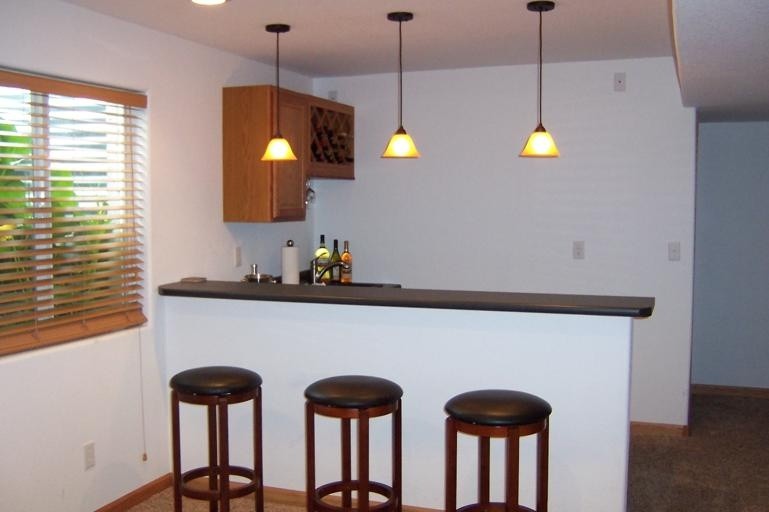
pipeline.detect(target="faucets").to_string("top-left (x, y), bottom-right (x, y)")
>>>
top-left (311, 253), bottom-right (351, 284)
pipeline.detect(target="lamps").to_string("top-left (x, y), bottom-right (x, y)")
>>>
top-left (518, 0), bottom-right (561, 158)
top-left (259, 24), bottom-right (297, 161)
top-left (380, 12), bottom-right (422, 158)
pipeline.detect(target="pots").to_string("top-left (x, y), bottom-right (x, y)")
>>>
top-left (240, 274), bottom-right (275, 284)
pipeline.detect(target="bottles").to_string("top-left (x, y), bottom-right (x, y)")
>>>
top-left (313, 234), bottom-right (330, 286)
top-left (339, 241), bottom-right (352, 284)
top-left (327, 239), bottom-right (341, 283)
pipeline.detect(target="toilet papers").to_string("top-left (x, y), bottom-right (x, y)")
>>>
top-left (282, 247), bottom-right (300, 285)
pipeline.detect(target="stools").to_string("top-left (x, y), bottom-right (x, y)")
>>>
top-left (445, 389), bottom-right (552, 512)
top-left (304, 375), bottom-right (402, 512)
top-left (169, 365), bottom-right (264, 512)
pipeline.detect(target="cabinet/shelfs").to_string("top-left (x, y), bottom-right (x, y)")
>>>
top-left (223, 84), bottom-right (354, 223)
